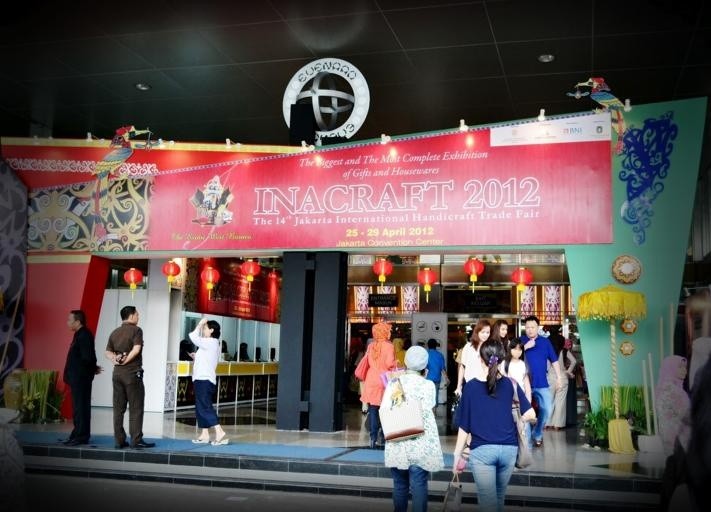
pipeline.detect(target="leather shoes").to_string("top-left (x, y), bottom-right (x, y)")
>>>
top-left (132, 440), bottom-right (156, 448)
top-left (115, 443), bottom-right (129, 449)
top-left (61, 439), bottom-right (81, 446)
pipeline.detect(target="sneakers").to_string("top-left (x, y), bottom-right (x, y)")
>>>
top-left (534, 440), bottom-right (542, 446)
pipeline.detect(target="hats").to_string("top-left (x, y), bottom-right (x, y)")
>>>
top-left (404, 346), bottom-right (429, 370)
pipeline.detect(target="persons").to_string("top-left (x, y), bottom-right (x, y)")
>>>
top-left (347, 315), bottom-right (580, 511)
top-left (219, 340), bottom-right (233, 360)
top-left (188, 318), bottom-right (231, 445)
top-left (63, 309), bottom-right (104, 445)
top-left (104, 306), bottom-right (156, 448)
top-left (181, 337), bottom-right (197, 361)
top-left (655, 290), bottom-right (711, 511)
top-left (233, 342), bottom-right (249, 361)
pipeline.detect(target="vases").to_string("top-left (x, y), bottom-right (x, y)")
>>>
top-left (3, 368), bottom-right (30, 411)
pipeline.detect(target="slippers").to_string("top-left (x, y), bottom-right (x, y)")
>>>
top-left (210, 439), bottom-right (229, 446)
top-left (192, 440), bottom-right (209, 444)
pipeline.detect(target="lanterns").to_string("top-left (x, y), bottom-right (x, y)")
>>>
top-left (418, 267), bottom-right (438, 303)
top-left (511, 267), bottom-right (533, 301)
top-left (465, 258), bottom-right (485, 293)
top-left (163, 260), bottom-right (180, 283)
top-left (200, 267), bottom-right (221, 290)
top-left (373, 258), bottom-right (394, 288)
top-left (124, 268), bottom-right (143, 291)
top-left (240, 259), bottom-right (262, 282)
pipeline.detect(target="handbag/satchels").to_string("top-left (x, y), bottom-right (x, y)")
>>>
top-left (442, 483), bottom-right (463, 511)
top-left (512, 408), bottom-right (532, 469)
top-left (377, 397), bottom-right (425, 442)
top-left (381, 368), bottom-right (405, 387)
top-left (354, 353), bottom-right (370, 380)
